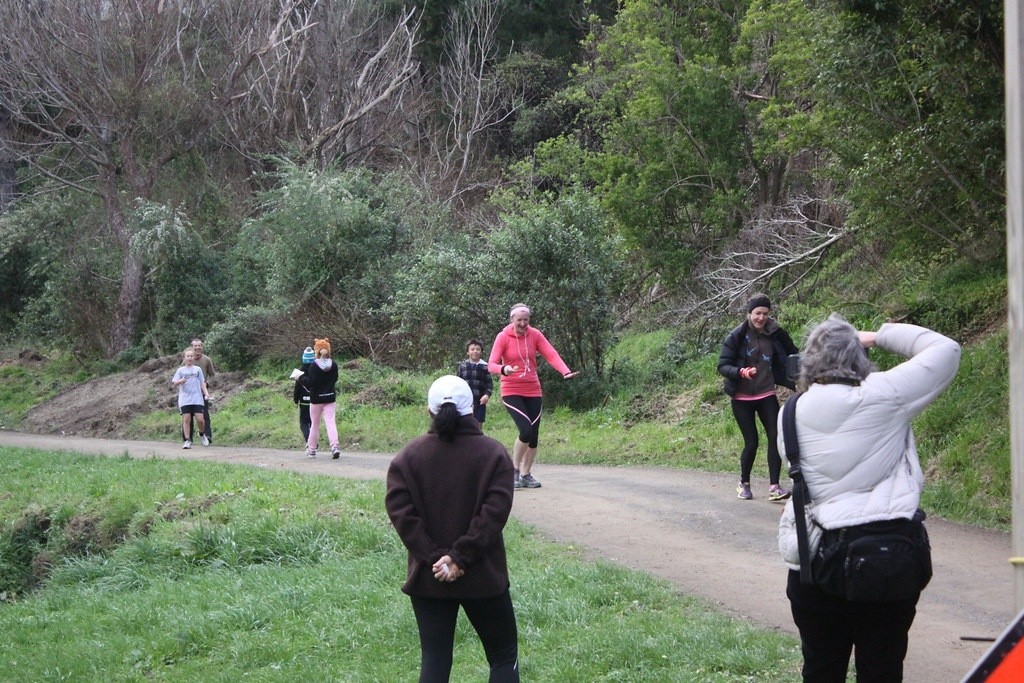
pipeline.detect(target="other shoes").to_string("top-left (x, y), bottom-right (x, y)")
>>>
top-left (206, 436), bottom-right (213, 444)
top-left (190, 440), bottom-right (194, 445)
top-left (305, 443), bottom-right (319, 452)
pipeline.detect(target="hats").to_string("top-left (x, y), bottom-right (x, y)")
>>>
top-left (427, 375), bottom-right (474, 418)
top-left (302, 346), bottom-right (318, 364)
top-left (314, 338), bottom-right (331, 359)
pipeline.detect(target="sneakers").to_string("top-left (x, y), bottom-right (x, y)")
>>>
top-left (197, 432), bottom-right (210, 447)
top-left (513, 468), bottom-right (521, 488)
top-left (331, 447), bottom-right (340, 460)
top-left (182, 440), bottom-right (193, 449)
top-left (736, 480), bottom-right (753, 500)
top-left (769, 484), bottom-right (792, 501)
top-left (305, 446), bottom-right (317, 458)
top-left (520, 473), bottom-right (541, 488)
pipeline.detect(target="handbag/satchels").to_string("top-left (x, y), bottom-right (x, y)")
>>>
top-left (806, 516), bottom-right (934, 631)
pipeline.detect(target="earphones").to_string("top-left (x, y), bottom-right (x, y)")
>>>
top-left (528, 367), bottom-right (530, 371)
top-left (519, 374), bottom-right (524, 377)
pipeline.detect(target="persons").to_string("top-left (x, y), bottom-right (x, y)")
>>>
top-left (384, 374), bottom-right (520, 683)
top-left (455, 338), bottom-right (493, 429)
top-left (294, 346), bottom-right (319, 454)
top-left (777, 311), bottom-right (961, 683)
top-left (717, 293), bottom-right (800, 502)
top-left (180, 339), bottom-right (216, 443)
top-left (172, 347), bottom-right (210, 449)
top-left (296, 338), bottom-right (340, 459)
top-left (488, 303), bottom-right (580, 490)
